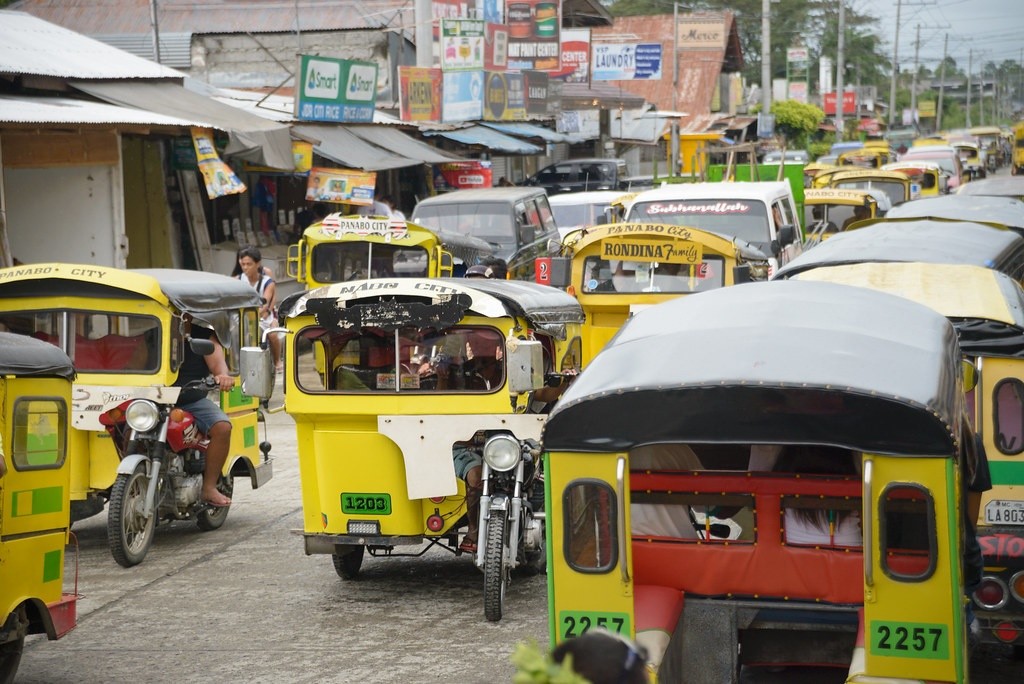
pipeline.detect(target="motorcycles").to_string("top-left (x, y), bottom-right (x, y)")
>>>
top-left (0, 124), bottom-right (1024, 684)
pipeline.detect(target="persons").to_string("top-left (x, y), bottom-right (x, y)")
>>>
top-left (629, 443), bottom-right (705, 539)
top-left (231, 245), bottom-right (282, 369)
top-left (746, 443), bottom-right (863, 545)
top-left (123, 323), bottom-right (236, 507)
top-left (435, 354), bottom-right (577, 553)
top-left (499, 177), bottom-right (515, 186)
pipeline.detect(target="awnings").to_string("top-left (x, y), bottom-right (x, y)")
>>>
top-left (423, 125), bottom-right (544, 155)
top-left (477, 122), bottom-right (585, 145)
top-left (290, 125), bottom-right (480, 172)
top-left (0, 81), bottom-right (295, 171)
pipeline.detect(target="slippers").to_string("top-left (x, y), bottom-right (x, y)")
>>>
top-left (201, 494), bottom-right (232, 507)
top-left (460, 535), bottom-right (478, 552)
